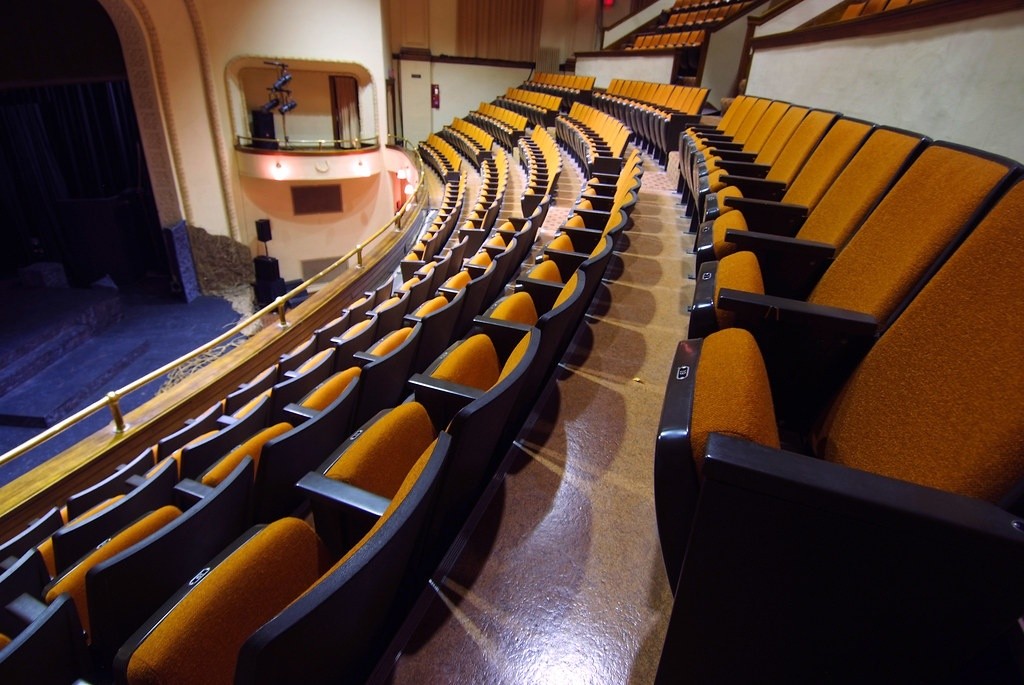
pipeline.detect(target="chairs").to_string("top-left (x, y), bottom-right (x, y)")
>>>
top-left (0, 0), bottom-right (1024, 685)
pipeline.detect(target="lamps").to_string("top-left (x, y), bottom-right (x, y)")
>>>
top-left (396, 166), bottom-right (409, 179)
top-left (357, 161), bottom-right (369, 177)
top-left (404, 181), bottom-right (418, 195)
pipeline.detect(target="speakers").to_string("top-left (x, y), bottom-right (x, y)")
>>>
top-left (252, 111), bottom-right (276, 148)
top-left (256, 219), bottom-right (272, 242)
top-left (254, 256), bottom-right (288, 312)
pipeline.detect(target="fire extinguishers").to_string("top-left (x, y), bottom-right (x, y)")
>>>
top-left (433, 86), bottom-right (439, 108)
